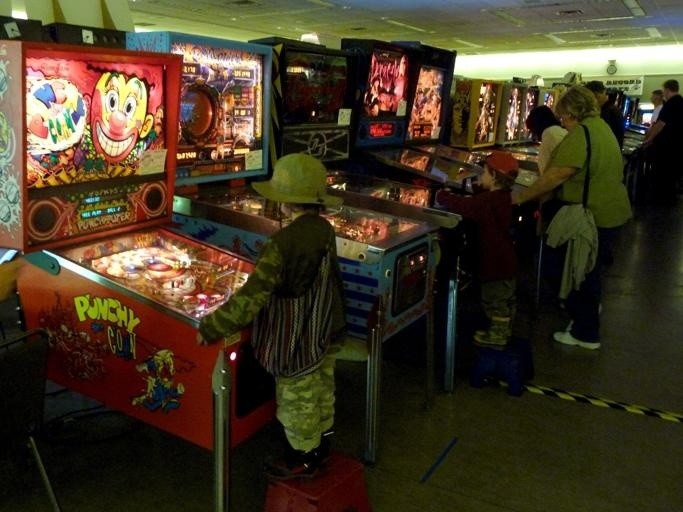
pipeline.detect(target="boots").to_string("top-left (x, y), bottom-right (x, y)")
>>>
top-left (473, 316), bottom-right (513, 351)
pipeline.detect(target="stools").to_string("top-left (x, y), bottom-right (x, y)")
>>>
top-left (264, 455), bottom-right (370, 510)
top-left (466, 318), bottom-right (535, 396)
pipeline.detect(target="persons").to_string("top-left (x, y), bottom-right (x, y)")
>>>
top-left (437, 152), bottom-right (521, 347)
top-left (512, 81), bottom-right (629, 350)
top-left (640, 80), bottom-right (681, 222)
top-left (195, 153), bottom-right (348, 464)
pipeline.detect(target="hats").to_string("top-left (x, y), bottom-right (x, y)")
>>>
top-left (475, 151), bottom-right (519, 180)
top-left (252, 152), bottom-right (344, 207)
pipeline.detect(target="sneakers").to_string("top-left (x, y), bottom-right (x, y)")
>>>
top-left (250, 434), bottom-right (331, 483)
top-left (552, 331), bottom-right (601, 350)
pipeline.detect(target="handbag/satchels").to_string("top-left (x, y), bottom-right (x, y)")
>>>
top-left (540, 197), bottom-right (586, 236)
